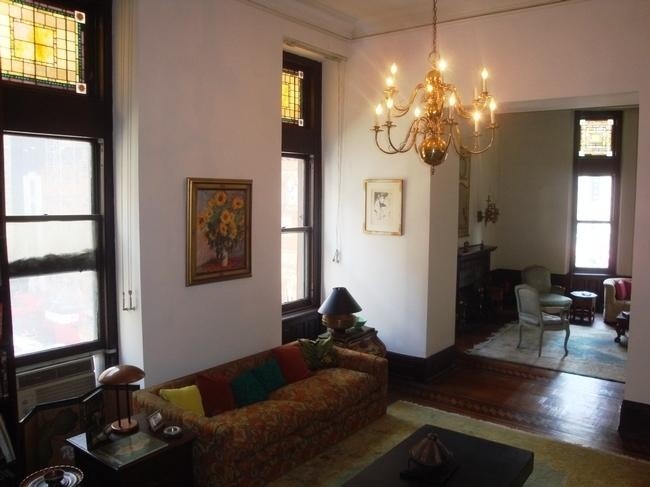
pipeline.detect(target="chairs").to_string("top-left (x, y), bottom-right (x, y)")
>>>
top-left (516, 285), bottom-right (571, 356)
top-left (522, 265), bottom-right (572, 319)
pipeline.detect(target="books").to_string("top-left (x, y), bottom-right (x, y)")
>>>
top-left (81, 388), bottom-right (114, 450)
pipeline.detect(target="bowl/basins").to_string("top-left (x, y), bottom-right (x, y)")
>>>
top-left (353, 317), bottom-right (367, 328)
top-left (42, 469), bottom-right (66, 487)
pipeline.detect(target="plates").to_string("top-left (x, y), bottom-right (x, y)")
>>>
top-left (26, 472), bottom-right (79, 487)
top-left (19, 465), bottom-right (84, 487)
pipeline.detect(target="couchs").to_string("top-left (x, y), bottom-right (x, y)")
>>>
top-left (133, 338), bottom-right (388, 486)
top-left (604, 277), bottom-right (632, 323)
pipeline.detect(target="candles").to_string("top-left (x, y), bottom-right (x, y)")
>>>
top-left (391, 62), bottom-right (398, 83)
top-left (428, 83), bottom-right (434, 99)
top-left (415, 107), bottom-right (421, 128)
top-left (387, 97), bottom-right (392, 123)
top-left (387, 77), bottom-right (393, 94)
top-left (473, 109), bottom-right (481, 133)
top-left (481, 67), bottom-right (490, 96)
top-left (488, 96), bottom-right (498, 125)
top-left (474, 83), bottom-right (480, 102)
top-left (449, 92), bottom-right (457, 121)
top-left (375, 102), bottom-right (383, 126)
top-left (438, 61), bottom-right (448, 85)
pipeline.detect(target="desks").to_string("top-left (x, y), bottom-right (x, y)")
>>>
top-left (570, 291), bottom-right (597, 326)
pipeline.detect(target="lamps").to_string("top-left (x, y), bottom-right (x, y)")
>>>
top-left (479, 195), bottom-right (500, 224)
top-left (371, 5), bottom-right (499, 173)
top-left (318, 287), bottom-right (361, 339)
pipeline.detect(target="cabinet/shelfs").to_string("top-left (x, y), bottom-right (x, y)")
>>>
top-left (458, 242), bottom-right (498, 322)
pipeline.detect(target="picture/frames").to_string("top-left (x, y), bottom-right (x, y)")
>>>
top-left (184, 178), bottom-right (251, 287)
top-left (363, 179), bottom-right (404, 238)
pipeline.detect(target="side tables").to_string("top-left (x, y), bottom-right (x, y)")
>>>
top-left (63, 409), bottom-right (197, 487)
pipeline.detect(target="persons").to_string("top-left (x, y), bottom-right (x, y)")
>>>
top-left (375, 194), bottom-right (389, 221)
